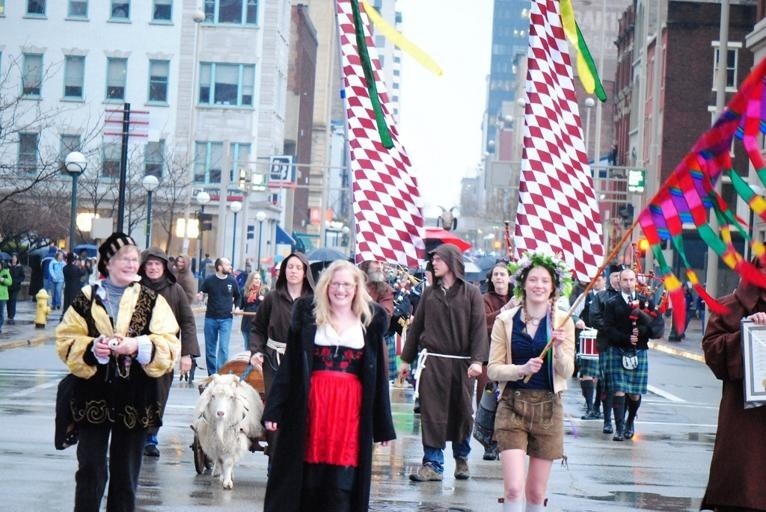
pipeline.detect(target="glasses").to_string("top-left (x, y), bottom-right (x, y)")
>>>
top-left (328, 281), bottom-right (357, 290)
top-left (430, 257), bottom-right (443, 262)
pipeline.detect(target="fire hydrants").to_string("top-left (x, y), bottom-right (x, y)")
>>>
top-left (35, 288), bottom-right (53, 329)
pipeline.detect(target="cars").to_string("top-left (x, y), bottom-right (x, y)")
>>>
top-left (73, 244), bottom-right (99, 258)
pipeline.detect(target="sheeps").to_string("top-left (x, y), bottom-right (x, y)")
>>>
top-left (195, 373), bottom-right (265, 489)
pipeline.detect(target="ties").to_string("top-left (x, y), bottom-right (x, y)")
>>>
top-left (628, 296), bottom-right (632, 302)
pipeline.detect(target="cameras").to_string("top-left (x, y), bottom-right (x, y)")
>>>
top-left (101, 337), bottom-right (120, 349)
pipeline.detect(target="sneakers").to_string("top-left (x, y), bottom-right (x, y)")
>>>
top-left (483, 447), bottom-right (498, 460)
top-left (144, 445), bottom-right (159, 457)
top-left (454, 459), bottom-right (469, 479)
top-left (409, 464), bottom-right (443, 481)
top-left (414, 398), bottom-right (421, 413)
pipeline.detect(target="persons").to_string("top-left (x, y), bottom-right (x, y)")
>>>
top-left (170, 252), bottom-right (279, 384)
top-left (400, 243), bottom-right (487, 482)
top-left (479, 273), bottom-right (491, 294)
top-left (473, 259), bottom-right (521, 460)
top-left (634, 281), bottom-right (708, 342)
top-left (488, 262), bottom-right (576, 510)
top-left (249, 251), bottom-right (316, 480)
top-left (136, 247), bottom-right (201, 456)
top-left (569, 273), bottom-right (608, 419)
top-left (260, 259), bottom-right (396, 511)
top-left (590, 265), bottom-right (624, 433)
top-left (699, 242), bottom-right (766, 510)
top-left (0, 246), bottom-right (101, 325)
top-left (356, 260), bottom-right (433, 416)
top-left (54, 233), bottom-right (180, 510)
top-left (606, 268), bottom-right (665, 440)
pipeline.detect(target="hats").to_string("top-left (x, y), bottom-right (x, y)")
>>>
top-left (610, 264), bottom-right (626, 273)
top-left (97, 232), bottom-right (136, 279)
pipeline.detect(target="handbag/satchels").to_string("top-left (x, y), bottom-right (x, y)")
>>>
top-left (473, 382), bottom-right (497, 448)
top-left (55, 373), bottom-right (80, 450)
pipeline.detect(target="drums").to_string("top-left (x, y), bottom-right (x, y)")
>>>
top-left (578, 328), bottom-right (599, 361)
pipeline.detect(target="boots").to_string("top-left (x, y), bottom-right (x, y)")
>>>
top-left (581, 379), bottom-right (642, 440)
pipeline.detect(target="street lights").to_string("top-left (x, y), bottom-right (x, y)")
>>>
top-left (325, 219), bottom-right (350, 247)
top-left (141, 175), bottom-right (161, 248)
top-left (228, 201), bottom-right (242, 270)
top-left (255, 211), bottom-right (266, 272)
top-left (64, 149), bottom-right (86, 260)
top-left (196, 192), bottom-right (212, 289)
top-left (584, 95), bottom-right (595, 159)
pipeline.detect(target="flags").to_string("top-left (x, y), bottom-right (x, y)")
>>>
top-left (516, 1), bottom-right (608, 281)
top-left (336, 1), bottom-right (426, 267)
